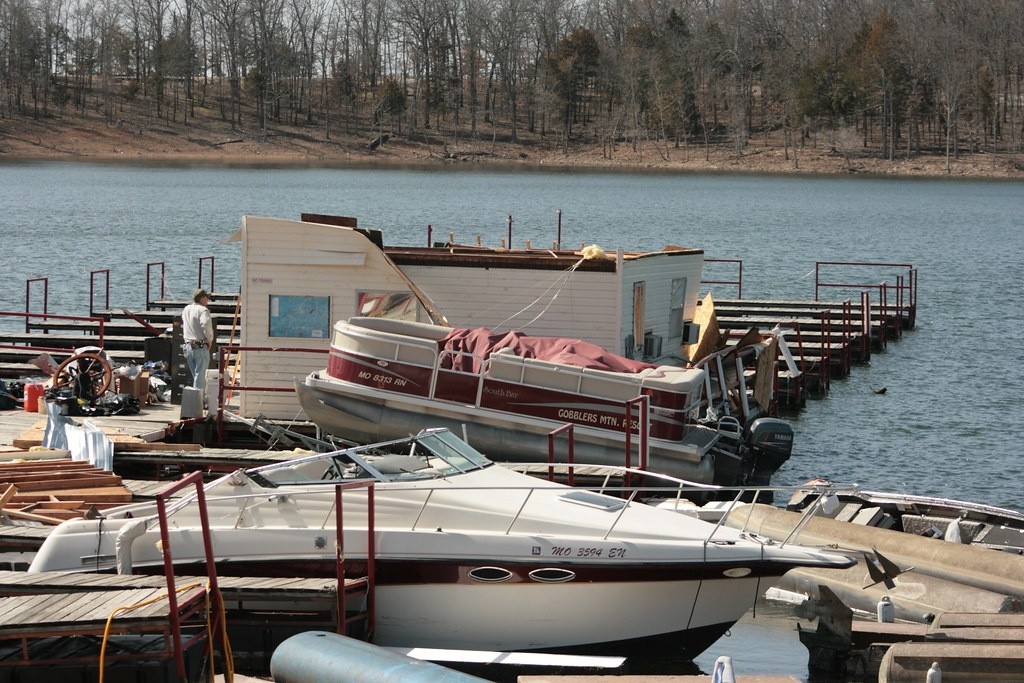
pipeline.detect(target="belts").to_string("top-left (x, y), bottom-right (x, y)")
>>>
top-left (184, 341), bottom-right (207, 345)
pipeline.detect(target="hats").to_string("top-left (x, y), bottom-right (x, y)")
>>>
top-left (193, 289), bottom-right (206, 301)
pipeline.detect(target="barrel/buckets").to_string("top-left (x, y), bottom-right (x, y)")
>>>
top-left (38, 396), bottom-right (46, 415)
top-left (24, 378), bottom-right (43, 411)
top-left (46, 399), bottom-right (61, 405)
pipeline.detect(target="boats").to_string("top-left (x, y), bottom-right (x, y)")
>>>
top-left (293, 317), bottom-right (794, 506)
top-left (26, 426), bottom-right (858, 683)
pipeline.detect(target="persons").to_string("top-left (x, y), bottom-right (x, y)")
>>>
top-left (182, 289), bottom-right (213, 410)
